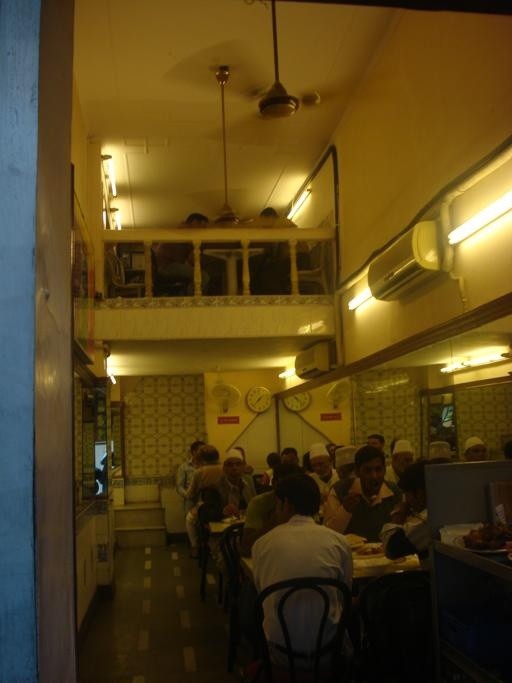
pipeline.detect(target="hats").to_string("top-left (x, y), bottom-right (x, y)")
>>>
top-left (309, 442), bottom-right (330, 458)
top-left (224, 448), bottom-right (243, 461)
top-left (393, 436), bottom-right (485, 461)
top-left (335, 446), bottom-right (360, 467)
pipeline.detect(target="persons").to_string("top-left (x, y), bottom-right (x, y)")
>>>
top-left (176, 435), bottom-right (511, 683)
top-left (249, 207), bottom-right (310, 295)
top-left (156, 214), bottom-right (211, 296)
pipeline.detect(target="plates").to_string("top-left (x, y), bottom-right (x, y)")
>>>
top-left (349, 543), bottom-right (385, 559)
top-left (452, 533), bottom-right (508, 553)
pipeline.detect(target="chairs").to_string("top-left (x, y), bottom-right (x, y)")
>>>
top-left (195, 472), bottom-right (431, 682)
top-left (297, 238), bottom-right (331, 297)
top-left (104, 242), bottom-right (144, 300)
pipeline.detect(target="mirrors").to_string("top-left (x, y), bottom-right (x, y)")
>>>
top-left (270, 288), bottom-right (511, 499)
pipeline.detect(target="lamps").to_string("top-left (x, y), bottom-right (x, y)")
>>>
top-left (447, 187), bottom-right (512, 255)
top-left (102, 149), bottom-right (120, 199)
top-left (286, 185), bottom-right (314, 223)
top-left (347, 288), bottom-right (373, 313)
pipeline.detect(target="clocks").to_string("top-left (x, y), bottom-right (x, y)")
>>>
top-left (283, 389), bottom-right (313, 413)
top-left (246, 384), bottom-right (273, 414)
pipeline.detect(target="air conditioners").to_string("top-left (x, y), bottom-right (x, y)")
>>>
top-left (367, 217), bottom-right (455, 303)
top-left (295, 338), bottom-right (338, 381)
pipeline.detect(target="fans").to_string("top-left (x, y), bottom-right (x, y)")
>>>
top-left (164, 0), bottom-right (391, 148)
top-left (124, 65), bottom-right (300, 234)
top-left (208, 364), bottom-right (243, 413)
